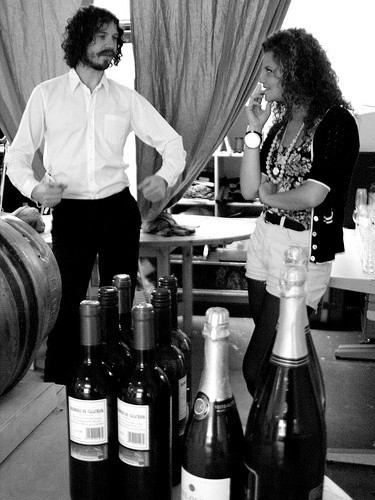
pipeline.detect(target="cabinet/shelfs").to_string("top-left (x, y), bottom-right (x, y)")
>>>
top-left (167, 148), bottom-right (254, 304)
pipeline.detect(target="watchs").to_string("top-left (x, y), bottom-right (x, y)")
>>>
top-left (243, 129), bottom-right (263, 148)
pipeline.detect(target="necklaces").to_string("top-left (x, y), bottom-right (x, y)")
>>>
top-left (266, 121), bottom-right (305, 185)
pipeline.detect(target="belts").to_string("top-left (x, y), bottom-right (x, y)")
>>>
top-left (261, 209), bottom-right (306, 231)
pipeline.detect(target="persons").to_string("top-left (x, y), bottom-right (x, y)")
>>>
top-left (6, 4), bottom-right (187, 393)
top-left (239, 30), bottom-right (359, 401)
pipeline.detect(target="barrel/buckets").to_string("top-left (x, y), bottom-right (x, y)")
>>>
top-left (0, 213), bottom-right (61, 399)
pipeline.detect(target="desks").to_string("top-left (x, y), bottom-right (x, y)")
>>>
top-left (140, 216), bottom-right (255, 337)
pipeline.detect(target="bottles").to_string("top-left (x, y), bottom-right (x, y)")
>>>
top-left (152, 289), bottom-right (187, 488)
top-left (67, 299), bottom-right (118, 500)
top-left (117, 303), bottom-right (171, 499)
top-left (98, 286), bottom-right (132, 378)
top-left (157, 276), bottom-right (192, 425)
top-left (113, 274), bottom-right (133, 346)
top-left (243, 266), bottom-right (327, 500)
top-left (254, 245), bottom-right (327, 416)
top-left (180, 306), bottom-right (243, 500)
